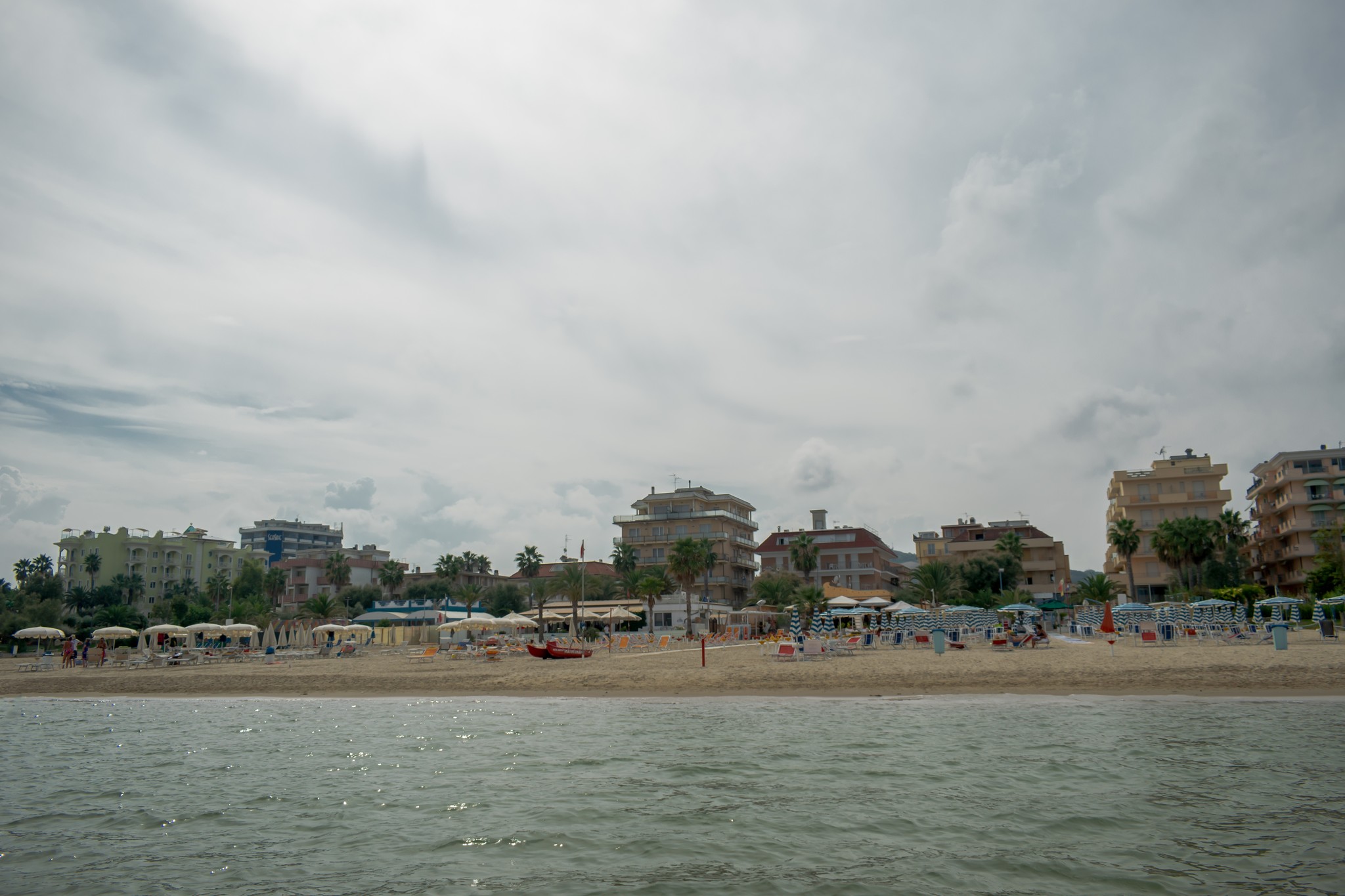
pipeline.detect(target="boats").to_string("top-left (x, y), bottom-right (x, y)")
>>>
top-left (545, 643), bottom-right (594, 658)
top-left (526, 645), bottom-right (555, 658)
top-left (471, 639), bottom-right (498, 646)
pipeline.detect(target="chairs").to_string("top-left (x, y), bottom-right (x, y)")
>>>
top-left (1318, 620), bottom-right (1339, 644)
top-left (379, 632), bottom-right (739, 664)
top-left (769, 624), bottom-right (1049, 663)
top-left (1069, 621), bottom-right (1274, 648)
top-left (88, 638), bottom-right (372, 670)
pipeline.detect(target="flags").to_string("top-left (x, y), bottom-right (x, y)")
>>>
top-left (581, 544), bottom-right (585, 558)
top-left (1059, 579), bottom-right (1070, 597)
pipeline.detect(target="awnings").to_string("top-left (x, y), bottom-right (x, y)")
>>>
top-left (1303, 477), bottom-right (1345, 512)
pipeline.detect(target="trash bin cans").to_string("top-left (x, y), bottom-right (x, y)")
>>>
top-left (551, 634), bottom-right (556, 641)
top-left (39, 648), bottom-right (44, 657)
top-left (1270, 624), bottom-right (1288, 650)
top-left (932, 629), bottom-right (945, 656)
top-left (266, 648), bottom-right (275, 664)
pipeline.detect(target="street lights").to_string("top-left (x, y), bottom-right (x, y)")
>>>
top-left (999, 567), bottom-right (1005, 597)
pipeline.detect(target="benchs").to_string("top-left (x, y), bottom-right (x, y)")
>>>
top-left (16, 663), bottom-right (64, 672)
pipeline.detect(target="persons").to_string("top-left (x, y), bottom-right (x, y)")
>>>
top-left (176, 645), bottom-right (188, 665)
top-left (743, 576), bottom-right (746, 580)
top-left (603, 624), bottom-right (610, 641)
top-left (995, 633), bottom-right (1028, 642)
top-left (61, 634), bottom-right (108, 669)
top-left (1031, 624), bottom-right (1047, 649)
top-left (758, 622), bottom-right (778, 634)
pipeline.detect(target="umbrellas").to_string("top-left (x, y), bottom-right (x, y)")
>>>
top-left (14, 622), bottom-right (376, 666)
top-left (783, 595), bottom-right (894, 663)
top-left (1038, 600), bottom-right (1073, 634)
top-left (881, 601), bottom-right (945, 648)
top-left (1252, 596), bottom-right (1306, 642)
top-left (1077, 600), bottom-right (1192, 657)
top-left (1190, 598), bottom-right (1247, 644)
top-left (389, 604), bottom-right (641, 653)
top-left (1311, 595), bottom-right (1326, 641)
top-left (996, 602), bottom-right (1041, 631)
top-left (944, 605), bottom-right (999, 641)
top-left (1318, 594), bottom-right (1345, 605)
